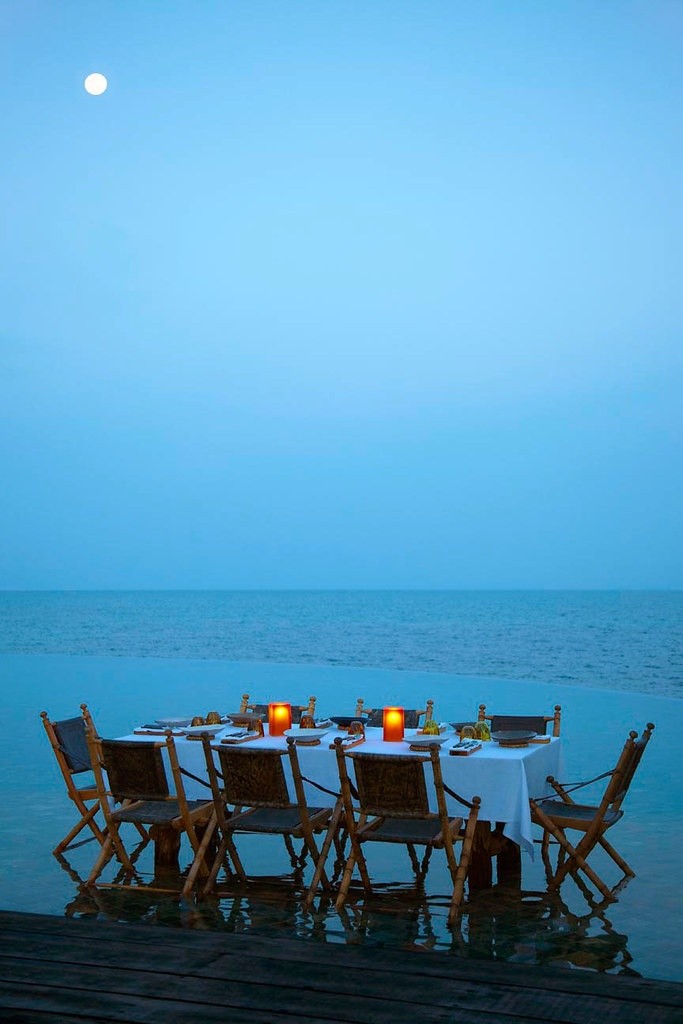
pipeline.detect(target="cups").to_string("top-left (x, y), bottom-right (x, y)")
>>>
top-left (300, 715), bottom-right (315, 728)
top-left (348, 721), bottom-right (365, 738)
top-left (191, 717), bottom-right (204, 726)
top-left (459, 726), bottom-right (476, 742)
top-left (423, 720), bottom-right (440, 735)
top-left (206, 712), bottom-right (221, 724)
top-left (474, 721), bottom-right (490, 740)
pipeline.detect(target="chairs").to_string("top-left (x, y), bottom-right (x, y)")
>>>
top-left (40, 694), bottom-right (655, 924)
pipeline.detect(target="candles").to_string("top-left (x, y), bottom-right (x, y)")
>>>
top-left (382, 707), bottom-right (405, 743)
top-left (267, 702), bottom-right (292, 736)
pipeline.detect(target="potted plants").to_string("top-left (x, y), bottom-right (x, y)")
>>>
top-left (416, 723), bottom-right (446, 731)
top-left (315, 719), bottom-right (332, 727)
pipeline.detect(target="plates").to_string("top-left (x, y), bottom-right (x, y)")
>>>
top-left (283, 728), bottom-right (331, 742)
top-left (227, 713), bottom-right (265, 723)
top-left (178, 725), bottom-right (225, 736)
top-left (402, 735), bottom-right (449, 746)
top-left (491, 730), bottom-right (536, 743)
top-left (329, 716), bottom-right (371, 726)
top-left (449, 722), bottom-right (476, 731)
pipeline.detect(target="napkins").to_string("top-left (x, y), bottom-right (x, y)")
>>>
top-left (532, 735), bottom-right (551, 739)
top-left (134, 724), bottom-right (183, 733)
top-left (450, 737), bottom-right (482, 752)
top-left (224, 731), bottom-right (259, 741)
top-left (336, 734), bottom-right (364, 746)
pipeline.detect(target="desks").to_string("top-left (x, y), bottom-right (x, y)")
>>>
top-left (110, 719), bottom-right (560, 934)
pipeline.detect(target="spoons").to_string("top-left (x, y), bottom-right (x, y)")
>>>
top-left (465, 741), bottom-right (477, 748)
top-left (238, 731), bottom-right (254, 737)
top-left (347, 734), bottom-right (360, 741)
